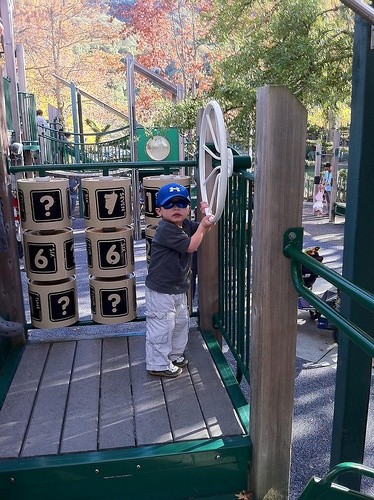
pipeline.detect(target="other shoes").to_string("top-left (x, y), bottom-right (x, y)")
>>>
top-left (173, 356), bottom-right (191, 369)
top-left (148, 365), bottom-right (183, 377)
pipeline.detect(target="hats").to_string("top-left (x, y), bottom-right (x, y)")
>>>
top-left (156, 182), bottom-right (191, 206)
top-left (324, 163), bottom-right (332, 166)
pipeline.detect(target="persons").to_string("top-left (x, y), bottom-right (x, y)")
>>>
top-left (322, 163), bottom-right (338, 217)
top-left (313, 176), bottom-right (329, 215)
top-left (36, 110), bottom-right (47, 126)
top-left (145, 182), bottom-right (217, 377)
top-left (63, 132), bottom-right (74, 148)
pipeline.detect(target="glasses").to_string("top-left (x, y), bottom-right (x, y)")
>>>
top-left (161, 200), bottom-right (188, 209)
